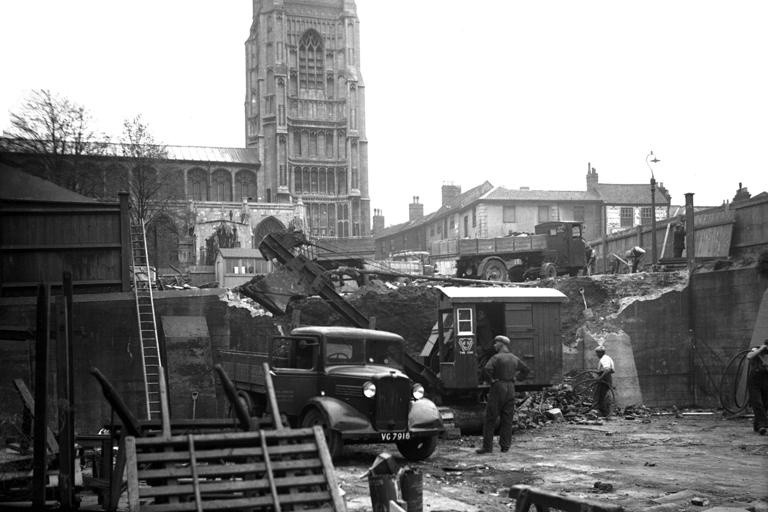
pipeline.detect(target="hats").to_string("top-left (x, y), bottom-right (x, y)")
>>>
top-left (494, 335), bottom-right (512, 345)
top-left (595, 345), bottom-right (606, 352)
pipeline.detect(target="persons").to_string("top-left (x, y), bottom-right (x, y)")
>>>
top-left (746, 338), bottom-right (767, 435)
top-left (582, 244), bottom-right (598, 277)
top-left (672, 223), bottom-right (687, 258)
top-left (475, 334), bottom-right (531, 453)
top-left (593, 344), bottom-right (615, 419)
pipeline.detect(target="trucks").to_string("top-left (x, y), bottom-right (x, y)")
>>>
top-left (217, 326), bottom-right (444, 460)
top-left (428, 220), bottom-right (597, 281)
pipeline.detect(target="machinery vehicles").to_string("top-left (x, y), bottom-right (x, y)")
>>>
top-left (232, 215), bottom-right (570, 435)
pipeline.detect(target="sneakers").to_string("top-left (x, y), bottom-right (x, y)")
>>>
top-left (500, 446), bottom-right (509, 453)
top-left (476, 446), bottom-right (490, 455)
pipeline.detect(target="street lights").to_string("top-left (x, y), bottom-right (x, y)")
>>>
top-left (646, 149), bottom-right (661, 263)
